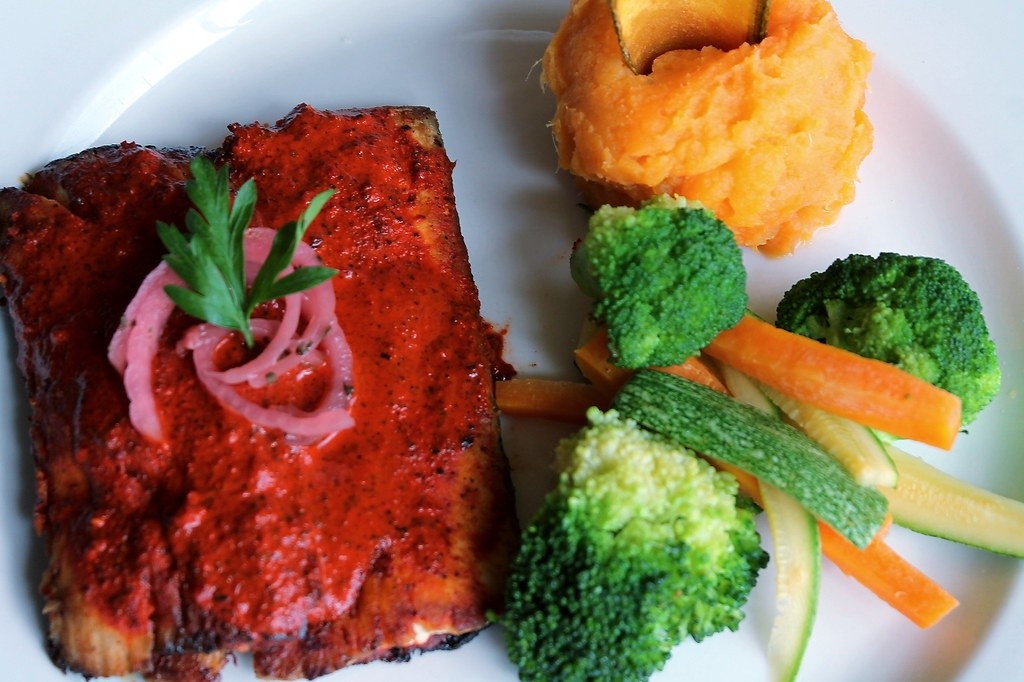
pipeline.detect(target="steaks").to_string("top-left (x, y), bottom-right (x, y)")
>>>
top-left (0, 100), bottom-right (528, 682)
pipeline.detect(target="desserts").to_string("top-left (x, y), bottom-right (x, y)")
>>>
top-left (540, 1), bottom-right (876, 261)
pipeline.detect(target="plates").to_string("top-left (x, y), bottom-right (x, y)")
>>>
top-left (0, 1), bottom-right (1024, 682)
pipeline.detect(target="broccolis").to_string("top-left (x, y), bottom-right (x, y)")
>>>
top-left (483, 191), bottom-right (999, 682)
